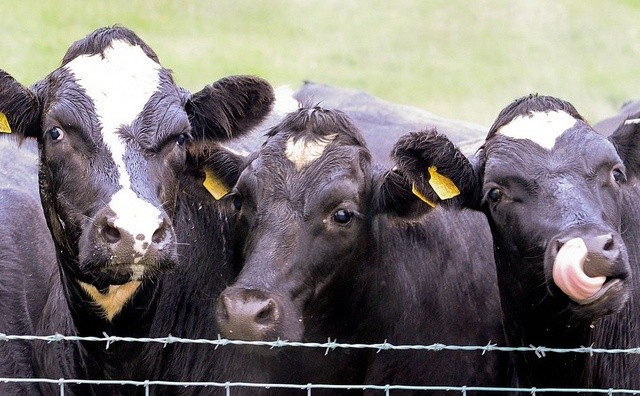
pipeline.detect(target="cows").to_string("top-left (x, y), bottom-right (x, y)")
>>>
top-left (0, 23), bottom-right (488, 395)
top-left (0, 134), bottom-right (59, 395)
top-left (389, 92), bottom-right (639, 395)
top-left (188, 96), bottom-right (522, 396)
